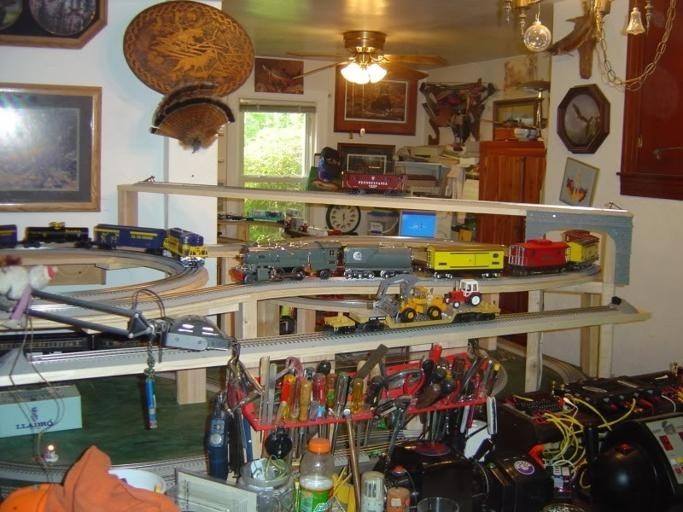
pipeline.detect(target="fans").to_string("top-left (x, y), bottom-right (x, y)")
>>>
top-left (286, 31), bottom-right (446, 81)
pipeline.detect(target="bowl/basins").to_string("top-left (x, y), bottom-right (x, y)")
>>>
top-left (107, 469), bottom-right (166, 495)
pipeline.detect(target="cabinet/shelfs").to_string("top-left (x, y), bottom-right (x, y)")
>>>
top-left (477, 140), bottom-right (547, 244)
top-left (616, 0), bottom-right (683, 200)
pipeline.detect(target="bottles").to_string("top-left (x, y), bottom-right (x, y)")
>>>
top-left (238, 458), bottom-right (294, 512)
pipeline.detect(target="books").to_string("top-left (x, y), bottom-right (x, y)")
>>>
top-left (394, 144), bottom-right (480, 200)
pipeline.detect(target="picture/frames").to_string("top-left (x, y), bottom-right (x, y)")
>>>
top-left (337, 144), bottom-right (396, 177)
top-left (491, 97), bottom-right (538, 141)
top-left (555, 84), bottom-right (611, 208)
top-left (0, 81), bottom-right (102, 212)
top-left (333, 65), bottom-right (418, 136)
top-left (0, 0), bottom-right (107, 51)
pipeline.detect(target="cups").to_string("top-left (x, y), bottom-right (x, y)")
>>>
top-left (296, 437), bottom-right (335, 512)
top-left (416, 496), bottom-right (459, 512)
top-left (385, 464), bottom-right (411, 512)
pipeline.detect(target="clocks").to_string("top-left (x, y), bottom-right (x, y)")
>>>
top-left (325, 204), bottom-right (361, 234)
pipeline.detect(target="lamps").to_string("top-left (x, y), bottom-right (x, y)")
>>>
top-left (339, 52), bottom-right (388, 85)
